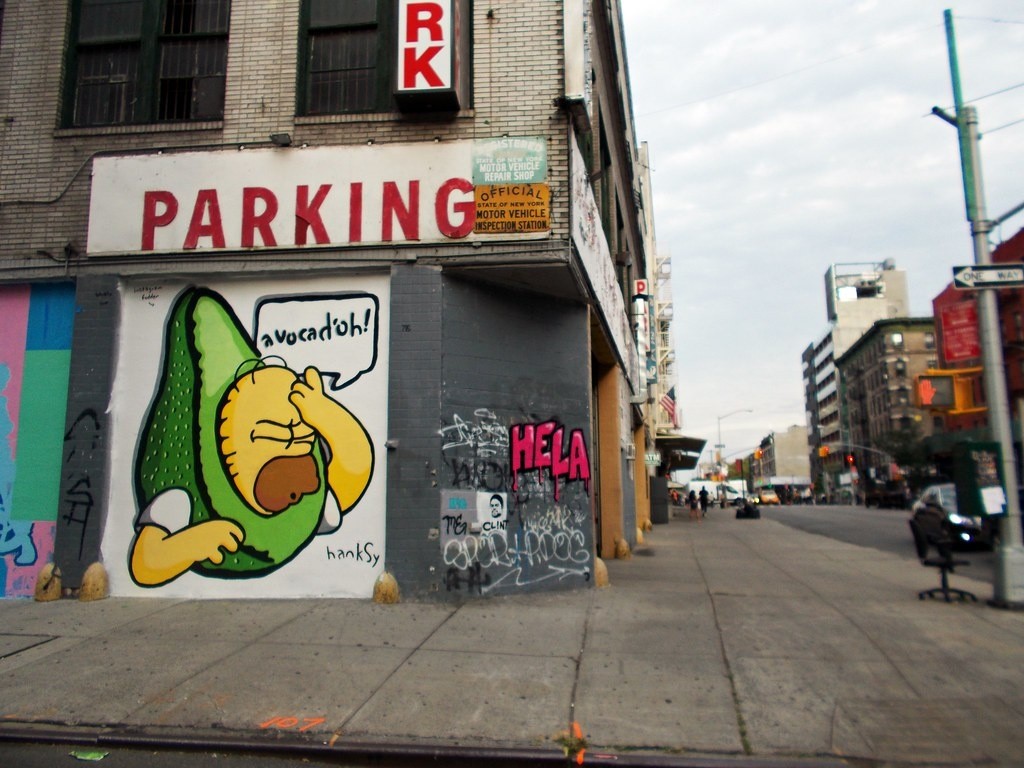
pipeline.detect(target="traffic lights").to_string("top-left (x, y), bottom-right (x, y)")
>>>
top-left (823, 448), bottom-right (829, 453)
top-left (911, 371), bottom-right (961, 410)
top-left (757, 449), bottom-right (764, 458)
top-left (849, 455), bottom-right (853, 463)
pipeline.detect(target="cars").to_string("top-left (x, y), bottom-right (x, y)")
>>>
top-left (912, 483), bottom-right (1008, 552)
top-left (758, 490), bottom-right (780, 506)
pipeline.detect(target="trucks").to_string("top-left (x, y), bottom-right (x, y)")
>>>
top-left (688, 481), bottom-right (743, 502)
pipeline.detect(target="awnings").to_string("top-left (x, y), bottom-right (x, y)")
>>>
top-left (670, 452), bottom-right (698, 469)
top-left (656, 432), bottom-right (707, 453)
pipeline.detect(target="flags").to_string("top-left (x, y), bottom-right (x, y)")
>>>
top-left (661, 387), bottom-right (677, 426)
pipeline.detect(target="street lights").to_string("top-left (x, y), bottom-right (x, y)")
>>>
top-left (717, 409), bottom-right (753, 470)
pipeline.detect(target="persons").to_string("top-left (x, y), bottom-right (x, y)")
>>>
top-left (700, 486), bottom-right (708, 517)
top-left (672, 490), bottom-right (678, 505)
top-left (689, 490), bottom-right (701, 521)
top-left (804, 488), bottom-right (812, 504)
top-left (743, 499), bottom-right (752, 517)
top-left (721, 495), bottom-right (725, 508)
top-left (709, 492), bottom-right (714, 507)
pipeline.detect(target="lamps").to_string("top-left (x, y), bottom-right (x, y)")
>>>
top-left (268, 133), bottom-right (292, 147)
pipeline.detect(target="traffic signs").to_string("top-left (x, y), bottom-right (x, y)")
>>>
top-left (952, 262), bottom-right (1024, 291)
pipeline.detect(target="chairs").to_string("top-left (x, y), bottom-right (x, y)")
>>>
top-left (909, 518), bottom-right (976, 601)
